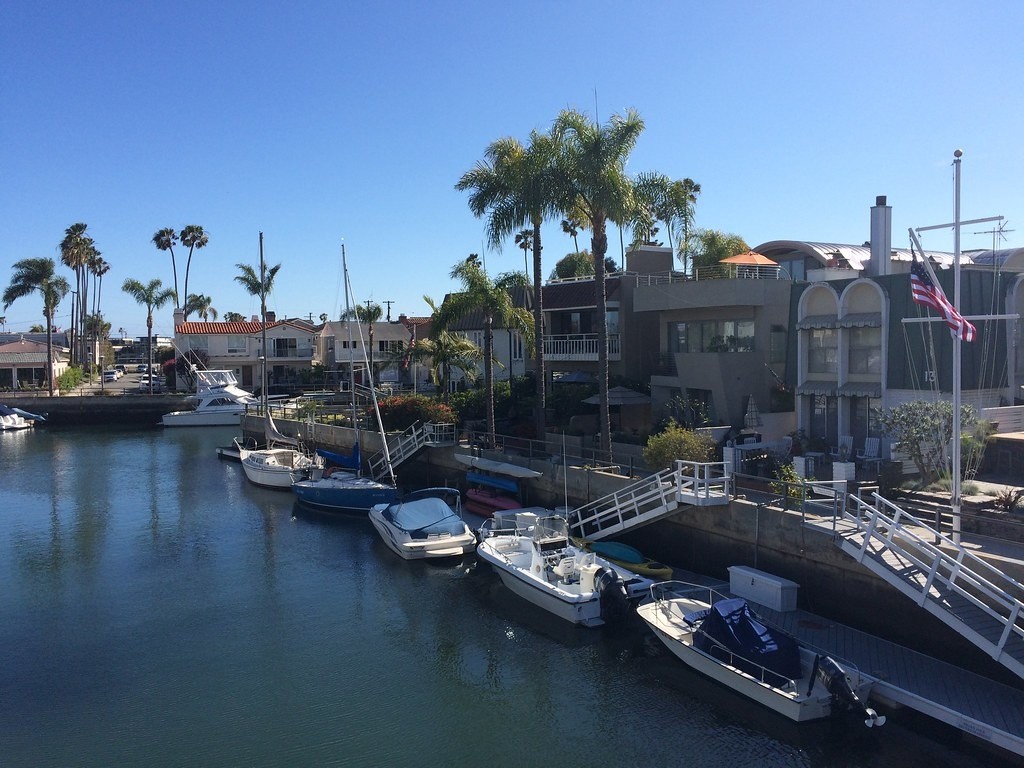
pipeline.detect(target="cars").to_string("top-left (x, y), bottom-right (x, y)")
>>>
top-left (137, 373), bottom-right (160, 394)
top-left (135, 364), bottom-right (156, 373)
top-left (104, 364), bottom-right (128, 382)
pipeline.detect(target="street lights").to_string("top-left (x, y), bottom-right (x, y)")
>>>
top-left (257, 355), bottom-right (265, 416)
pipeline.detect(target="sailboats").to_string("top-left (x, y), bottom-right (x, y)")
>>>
top-left (285, 242), bottom-right (404, 520)
top-left (231, 228), bottom-right (326, 492)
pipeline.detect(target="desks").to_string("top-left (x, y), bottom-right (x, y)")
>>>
top-left (805, 451), bottom-right (826, 467)
top-left (989, 431), bottom-right (1024, 473)
top-left (735, 442), bottom-right (771, 469)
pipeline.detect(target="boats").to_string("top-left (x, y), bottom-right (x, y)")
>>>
top-left (465, 487), bottom-right (522, 510)
top-left (367, 484), bottom-right (477, 564)
top-left (568, 534), bottom-right (674, 581)
top-left (452, 453), bottom-right (544, 479)
top-left (634, 577), bottom-right (889, 729)
top-left (466, 471), bottom-right (519, 494)
top-left (160, 336), bottom-right (282, 428)
top-left (476, 507), bottom-right (656, 629)
top-left (464, 501), bottom-right (498, 518)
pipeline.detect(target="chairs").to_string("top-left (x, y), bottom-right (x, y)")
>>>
top-left (855, 438), bottom-right (880, 472)
top-left (829, 436), bottom-right (854, 462)
top-left (726, 436), bottom-right (793, 472)
